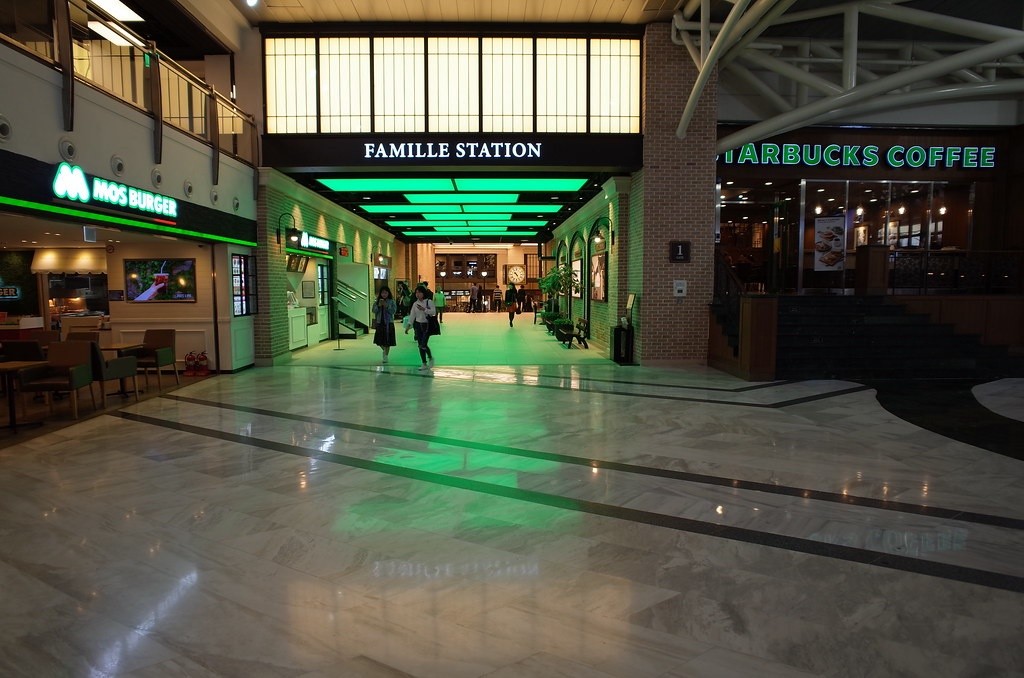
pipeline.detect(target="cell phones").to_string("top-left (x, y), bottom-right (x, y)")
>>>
top-left (417, 304), bottom-right (421, 307)
top-left (380, 299), bottom-right (384, 304)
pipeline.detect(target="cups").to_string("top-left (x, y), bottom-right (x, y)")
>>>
top-left (833, 237), bottom-right (841, 247)
top-left (154, 273), bottom-right (169, 295)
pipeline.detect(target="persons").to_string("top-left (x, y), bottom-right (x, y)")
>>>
top-left (469, 282), bottom-right (552, 328)
top-left (133, 282), bottom-right (164, 301)
top-left (372, 281), bottom-right (446, 371)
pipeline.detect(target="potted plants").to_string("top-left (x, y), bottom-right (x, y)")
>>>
top-left (537, 263), bottom-right (580, 341)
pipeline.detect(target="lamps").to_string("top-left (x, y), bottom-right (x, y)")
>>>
top-left (371, 245), bottom-right (383, 262)
top-left (595, 216), bottom-right (614, 245)
top-left (276, 213), bottom-right (298, 244)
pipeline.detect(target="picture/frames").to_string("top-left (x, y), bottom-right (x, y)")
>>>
top-left (302, 281), bottom-right (315, 298)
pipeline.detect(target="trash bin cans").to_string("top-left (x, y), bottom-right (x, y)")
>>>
top-left (610, 324), bottom-right (634, 363)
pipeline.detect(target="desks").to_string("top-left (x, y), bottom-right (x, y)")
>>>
top-left (0, 361), bottom-right (48, 433)
top-left (288, 307), bottom-right (308, 350)
top-left (99, 343), bottom-right (145, 397)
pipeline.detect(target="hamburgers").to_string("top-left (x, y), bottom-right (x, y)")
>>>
top-left (824, 231), bottom-right (833, 237)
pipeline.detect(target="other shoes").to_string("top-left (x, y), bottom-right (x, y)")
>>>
top-left (428, 359), bottom-right (433, 368)
top-left (419, 363), bottom-right (428, 370)
top-left (384, 356), bottom-right (388, 363)
top-left (383, 350), bottom-right (385, 357)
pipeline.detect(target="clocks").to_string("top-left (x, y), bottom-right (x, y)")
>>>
top-left (505, 265), bottom-right (527, 285)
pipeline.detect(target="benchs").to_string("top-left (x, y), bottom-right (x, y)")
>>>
top-left (559, 317), bottom-right (589, 348)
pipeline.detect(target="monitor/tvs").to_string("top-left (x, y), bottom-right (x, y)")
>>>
top-left (123, 258), bottom-right (197, 303)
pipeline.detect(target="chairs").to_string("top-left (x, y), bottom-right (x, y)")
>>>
top-left (0, 329), bottom-right (179, 421)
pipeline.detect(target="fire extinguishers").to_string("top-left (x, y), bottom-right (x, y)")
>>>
top-left (197, 350), bottom-right (208, 372)
top-left (184, 351), bottom-right (196, 373)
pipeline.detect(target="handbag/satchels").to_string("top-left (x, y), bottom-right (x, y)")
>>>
top-left (372, 319), bottom-right (377, 329)
top-left (426, 316), bottom-right (440, 335)
top-left (506, 302), bottom-right (513, 306)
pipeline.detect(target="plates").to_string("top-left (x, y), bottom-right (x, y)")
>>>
top-left (815, 240), bottom-right (832, 251)
top-left (819, 251), bottom-right (844, 266)
top-left (819, 230), bottom-right (833, 238)
top-left (831, 227), bottom-right (844, 234)
